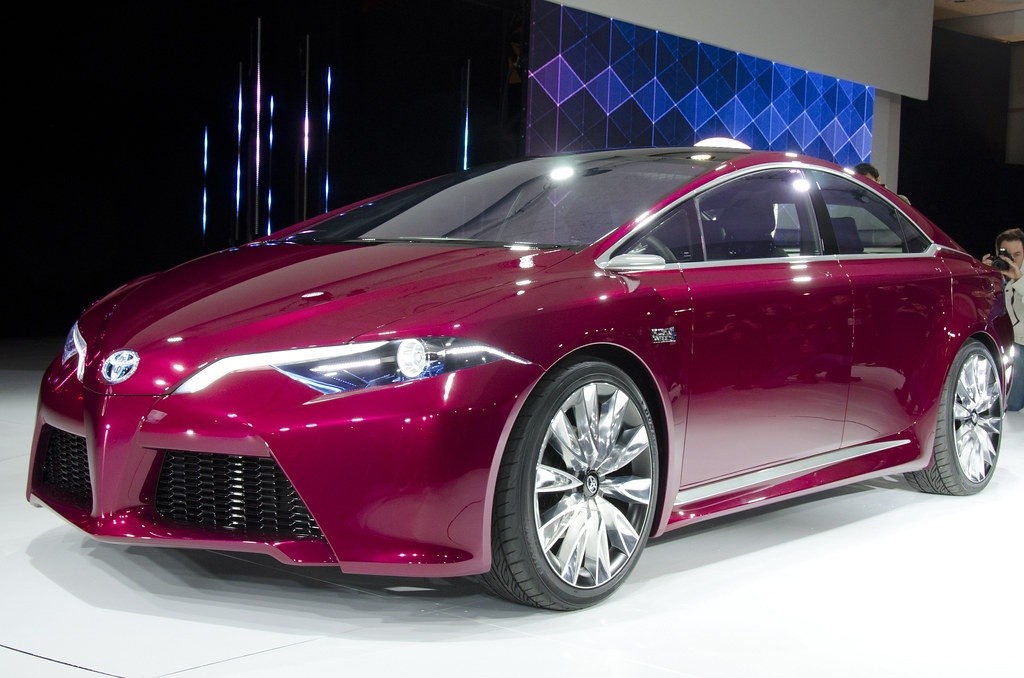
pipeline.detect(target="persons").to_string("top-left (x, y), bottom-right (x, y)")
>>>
top-left (982, 229), bottom-right (1024, 411)
top-left (854, 162), bottom-right (879, 182)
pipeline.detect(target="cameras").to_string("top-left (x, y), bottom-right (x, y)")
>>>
top-left (987, 248), bottom-right (1014, 270)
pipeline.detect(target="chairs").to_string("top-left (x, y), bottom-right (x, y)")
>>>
top-left (831, 217), bottom-right (864, 253)
top-left (718, 198), bottom-right (789, 258)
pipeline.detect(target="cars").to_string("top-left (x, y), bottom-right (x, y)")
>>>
top-left (25, 147), bottom-right (1015, 613)
